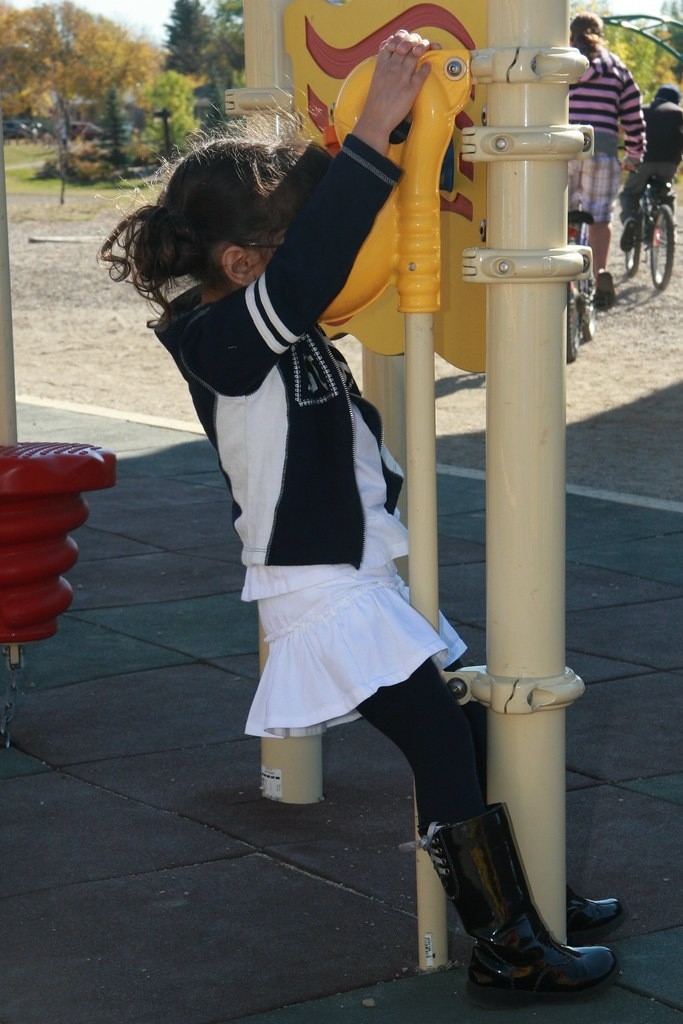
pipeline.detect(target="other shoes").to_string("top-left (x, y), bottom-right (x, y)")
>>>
top-left (593, 272), bottom-right (616, 311)
top-left (620, 216), bottom-right (638, 253)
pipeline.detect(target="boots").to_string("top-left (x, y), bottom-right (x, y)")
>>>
top-left (418, 804), bottom-right (625, 1009)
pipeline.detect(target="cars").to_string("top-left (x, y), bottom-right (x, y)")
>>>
top-left (4, 119), bottom-right (43, 140)
top-left (57, 121), bottom-right (111, 140)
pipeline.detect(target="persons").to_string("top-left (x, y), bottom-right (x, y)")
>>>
top-left (131, 28), bottom-right (624, 1003)
top-left (566, 12), bottom-right (646, 311)
top-left (618, 82), bottom-right (683, 253)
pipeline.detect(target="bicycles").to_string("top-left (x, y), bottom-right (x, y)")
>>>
top-left (621, 164), bottom-right (676, 291)
top-left (567, 141), bottom-right (627, 364)
